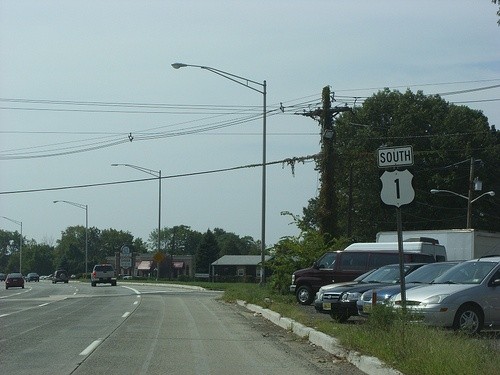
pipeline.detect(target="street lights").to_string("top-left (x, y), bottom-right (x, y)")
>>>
top-left (172, 61), bottom-right (268, 285)
top-left (110, 163), bottom-right (162, 281)
top-left (430, 188), bottom-right (495, 228)
top-left (53, 200), bottom-right (89, 280)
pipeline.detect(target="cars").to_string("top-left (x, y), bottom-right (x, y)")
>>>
top-left (24, 273), bottom-right (40, 282)
top-left (0, 273), bottom-right (78, 281)
top-left (356, 260), bottom-right (466, 316)
top-left (313, 262), bottom-right (427, 322)
top-left (388, 255), bottom-right (500, 336)
top-left (5, 273), bottom-right (25, 290)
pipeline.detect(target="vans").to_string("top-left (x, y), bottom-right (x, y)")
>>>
top-left (291, 250), bottom-right (435, 306)
top-left (331, 237), bottom-right (448, 266)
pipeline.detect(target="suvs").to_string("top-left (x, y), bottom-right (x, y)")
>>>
top-left (90, 264), bottom-right (117, 287)
top-left (51, 270), bottom-right (69, 284)
